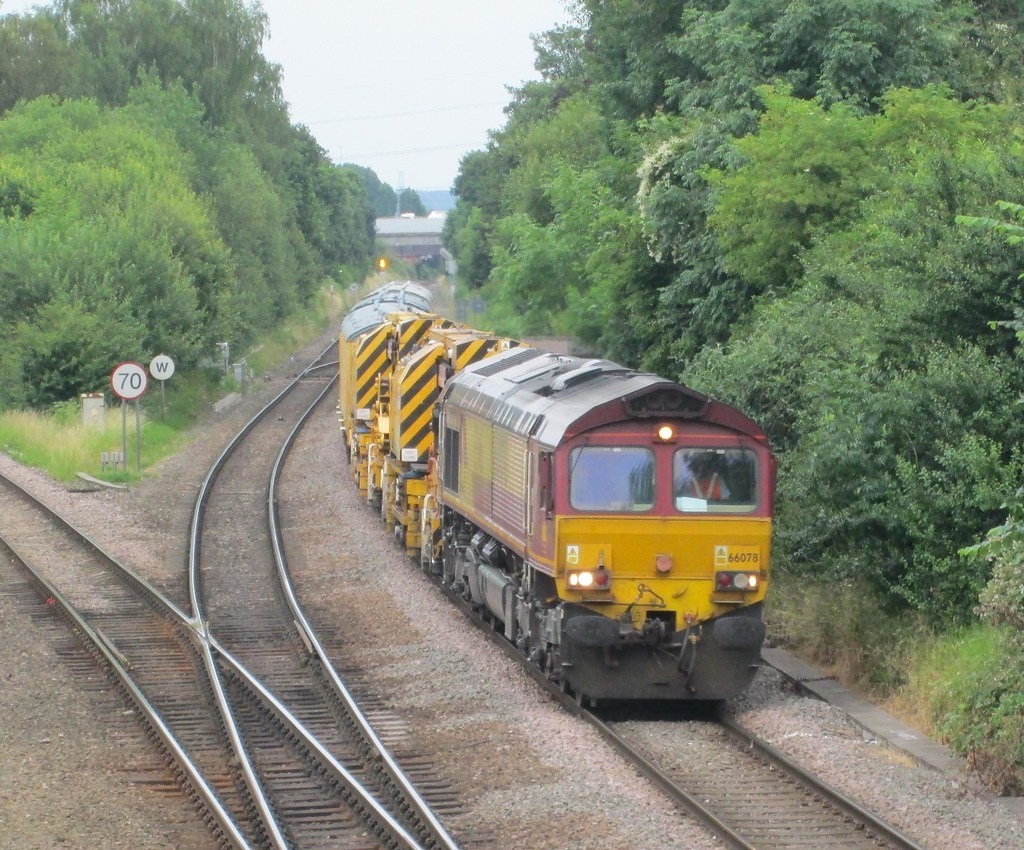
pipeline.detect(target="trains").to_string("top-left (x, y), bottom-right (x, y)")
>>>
top-left (339, 280), bottom-right (776, 708)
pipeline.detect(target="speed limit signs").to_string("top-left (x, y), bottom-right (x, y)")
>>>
top-left (111, 362), bottom-right (148, 400)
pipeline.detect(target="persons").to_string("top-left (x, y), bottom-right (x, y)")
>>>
top-left (680, 457), bottom-right (731, 499)
